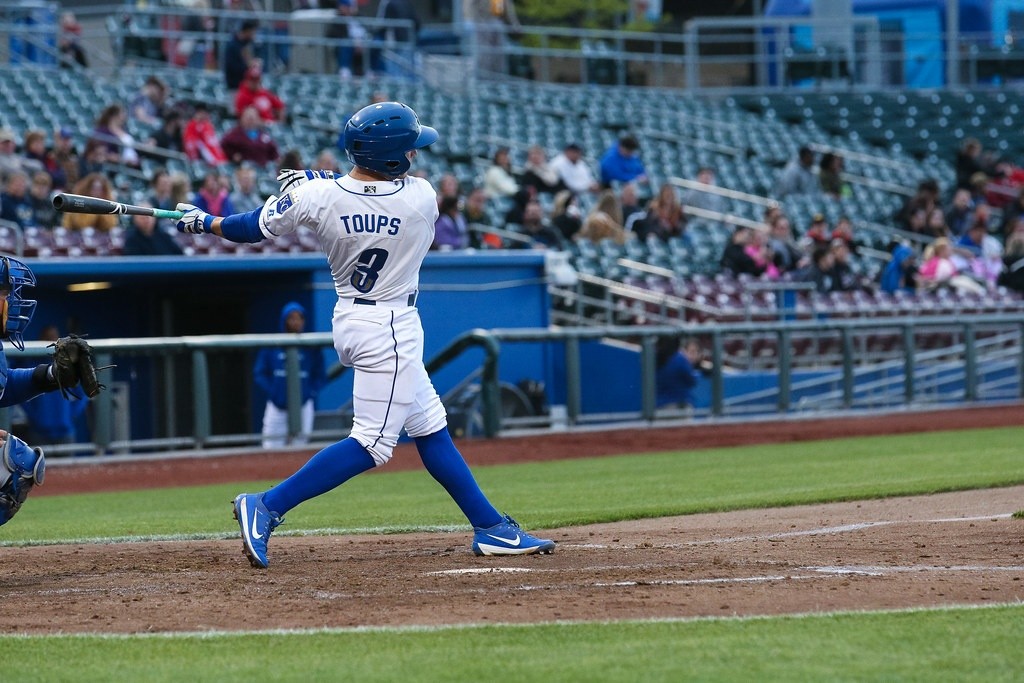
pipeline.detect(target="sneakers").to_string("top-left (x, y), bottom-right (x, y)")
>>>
top-left (230, 492), bottom-right (286, 569)
top-left (470, 512), bottom-right (556, 556)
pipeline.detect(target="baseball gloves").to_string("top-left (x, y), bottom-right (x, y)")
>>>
top-left (50, 334), bottom-right (102, 400)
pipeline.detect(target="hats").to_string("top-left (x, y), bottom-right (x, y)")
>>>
top-left (811, 213), bottom-right (827, 225)
top-left (487, 143), bottom-right (511, 158)
top-left (618, 130), bottom-right (641, 149)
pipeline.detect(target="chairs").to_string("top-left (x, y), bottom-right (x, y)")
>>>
top-left (0, 62), bottom-right (1024, 377)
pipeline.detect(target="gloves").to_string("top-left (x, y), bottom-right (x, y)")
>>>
top-left (168, 202), bottom-right (210, 234)
top-left (277, 169), bottom-right (334, 193)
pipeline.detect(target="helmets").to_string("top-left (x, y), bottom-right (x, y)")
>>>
top-left (343, 101), bottom-right (439, 178)
top-left (0, 255), bottom-right (38, 352)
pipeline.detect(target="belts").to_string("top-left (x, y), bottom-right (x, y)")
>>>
top-left (354, 289), bottom-right (417, 306)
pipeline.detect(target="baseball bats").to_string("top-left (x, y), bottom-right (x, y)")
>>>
top-left (52, 190), bottom-right (185, 219)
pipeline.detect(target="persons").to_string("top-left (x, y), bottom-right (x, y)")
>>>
top-left (0, 0), bottom-right (1024, 300)
top-left (253, 302), bottom-right (327, 451)
top-left (656, 336), bottom-right (702, 420)
top-left (169, 100), bottom-right (556, 570)
top-left (24, 325), bottom-right (89, 457)
top-left (0, 254), bottom-right (117, 527)
